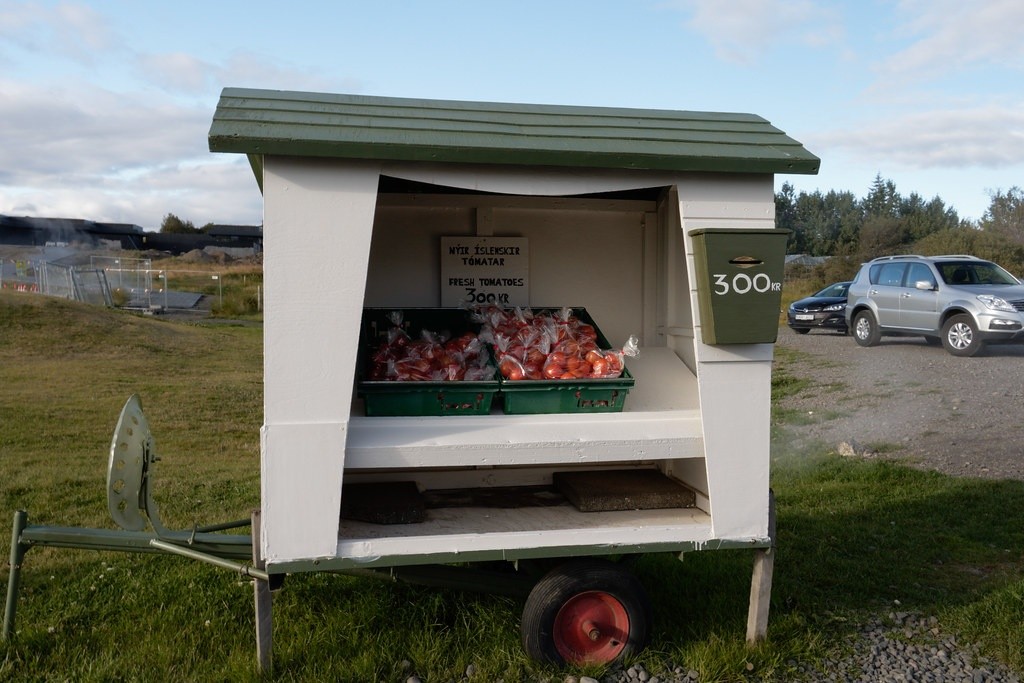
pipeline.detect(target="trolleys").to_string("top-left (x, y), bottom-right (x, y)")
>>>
top-left (0, 86), bottom-right (822, 676)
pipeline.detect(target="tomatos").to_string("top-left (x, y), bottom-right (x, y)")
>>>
top-left (365, 317), bottom-right (623, 382)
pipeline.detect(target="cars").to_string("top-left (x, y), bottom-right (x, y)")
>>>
top-left (786, 281), bottom-right (853, 335)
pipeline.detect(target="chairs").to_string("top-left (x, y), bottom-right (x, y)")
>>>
top-left (952, 267), bottom-right (975, 284)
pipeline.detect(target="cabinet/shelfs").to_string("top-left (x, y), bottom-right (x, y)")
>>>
top-left (337, 347), bottom-right (711, 542)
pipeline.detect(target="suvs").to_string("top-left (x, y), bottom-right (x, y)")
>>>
top-left (843, 254), bottom-right (1024, 357)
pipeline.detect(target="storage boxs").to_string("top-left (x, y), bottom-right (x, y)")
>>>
top-left (356, 306), bottom-right (499, 413)
top-left (477, 307), bottom-right (635, 415)
